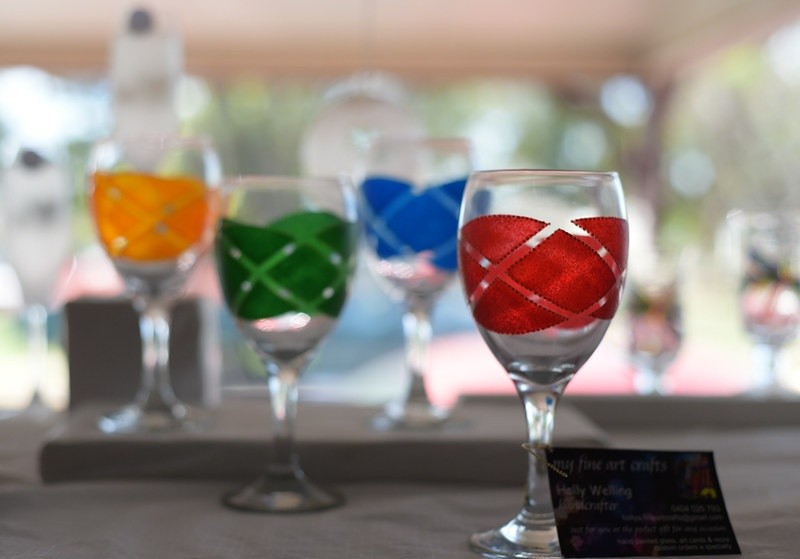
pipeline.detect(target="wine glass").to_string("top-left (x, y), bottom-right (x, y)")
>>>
top-left (615, 277), bottom-right (682, 397)
top-left (350, 135), bottom-right (475, 432)
top-left (81, 130), bottom-right (222, 438)
top-left (212, 174), bottom-right (355, 514)
top-left (0, 147), bottom-right (90, 425)
top-left (456, 167), bottom-right (630, 559)
top-left (737, 271), bottom-right (800, 400)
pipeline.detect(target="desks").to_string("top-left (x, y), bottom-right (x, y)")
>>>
top-left (0, 387), bottom-right (800, 559)
